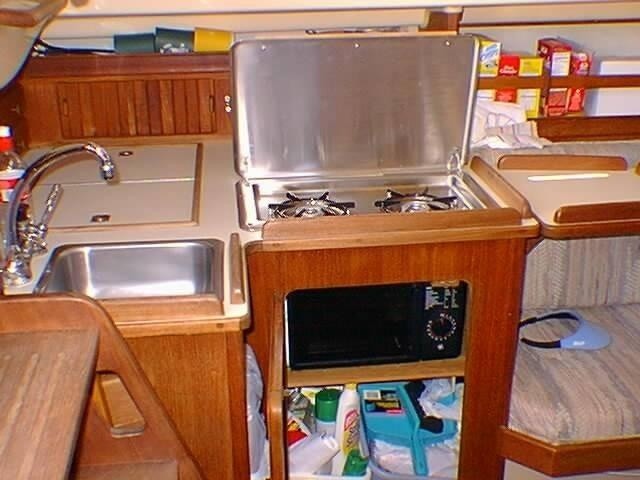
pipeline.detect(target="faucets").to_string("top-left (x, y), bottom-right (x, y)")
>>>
top-left (7, 141), bottom-right (116, 288)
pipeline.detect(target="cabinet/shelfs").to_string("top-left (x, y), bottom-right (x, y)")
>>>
top-left (0, 297), bottom-right (210, 480)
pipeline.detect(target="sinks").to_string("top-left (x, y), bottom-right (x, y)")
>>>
top-left (32, 238), bottom-right (226, 304)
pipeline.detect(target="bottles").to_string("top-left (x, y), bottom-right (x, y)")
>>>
top-left (0, 136), bottom-right (33, 273)
top-left (315, 382), bottom-right (361, 454)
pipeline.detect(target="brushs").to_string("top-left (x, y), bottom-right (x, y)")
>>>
top-left (397, 380), bottom-right (444, 476)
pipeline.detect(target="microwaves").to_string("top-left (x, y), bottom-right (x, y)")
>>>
top-left (281, 281), bottom-right (470, 371)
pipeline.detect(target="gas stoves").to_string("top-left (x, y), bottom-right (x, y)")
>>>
top-left (239, 169), bottom-right (502, 232)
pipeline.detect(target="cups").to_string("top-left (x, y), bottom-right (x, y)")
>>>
top-left (112, 25), bottom-right (236, 54)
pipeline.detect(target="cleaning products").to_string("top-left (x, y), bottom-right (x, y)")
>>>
top-left (333, 381), bottom-right (361, 475)
top-left (0, 123), bottom-right (33, 262)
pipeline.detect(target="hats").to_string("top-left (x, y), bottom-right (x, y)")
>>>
top-left (519, 309), bottom-right (610, 350)
